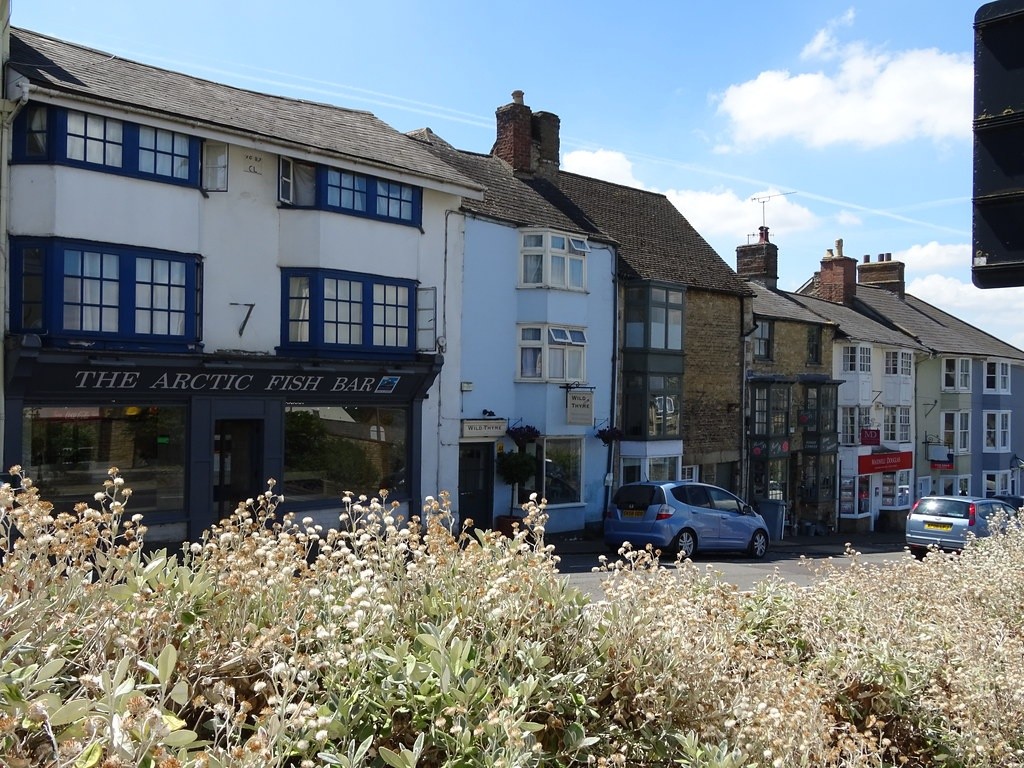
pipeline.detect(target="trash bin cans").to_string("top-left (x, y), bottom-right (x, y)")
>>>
top-left (496, 515), bottom-right (523, 555)
top-left (754, 499), bottom-right (786, 541)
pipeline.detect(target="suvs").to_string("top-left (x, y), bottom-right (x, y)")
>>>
top-left (905, 496), bottom-right (1018, 562)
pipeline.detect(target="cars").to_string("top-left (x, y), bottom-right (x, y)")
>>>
top-left (991, 493), bottom-right (1024, 511)
top-left (604, 480), bottom-right (770, 561)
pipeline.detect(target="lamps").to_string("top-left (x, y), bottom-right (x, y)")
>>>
top-left (482, 409), bottom-right (496, 416)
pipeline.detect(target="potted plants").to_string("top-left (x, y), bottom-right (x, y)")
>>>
top-left (496, 425), bottom-right (540, 539)
top-left (594, 427), bottom-right (626, 447)
